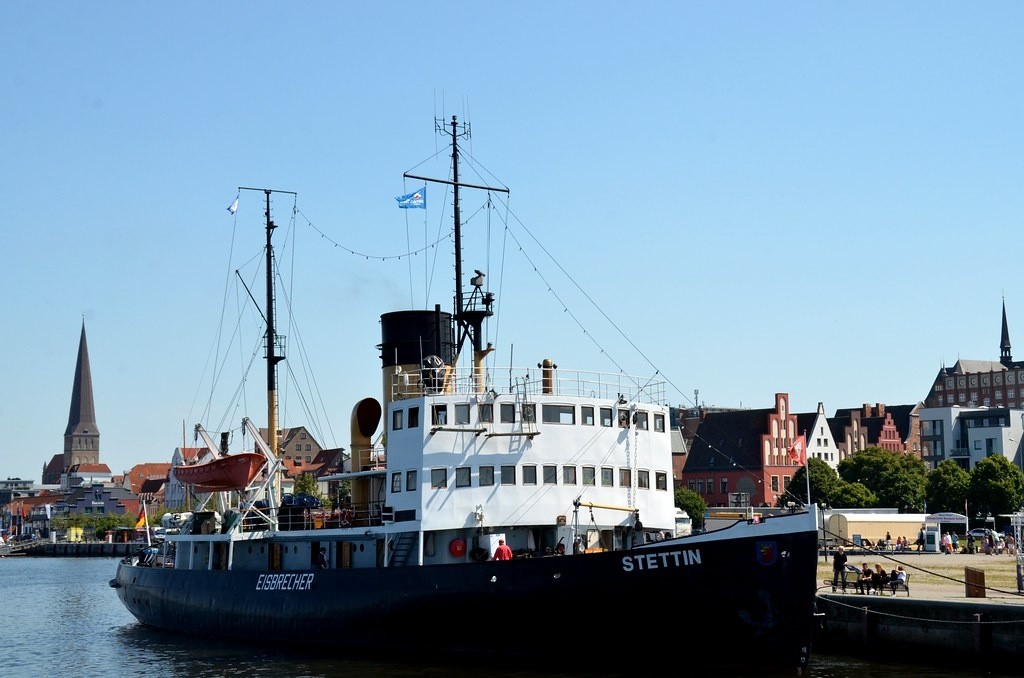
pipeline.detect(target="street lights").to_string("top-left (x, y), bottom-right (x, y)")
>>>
top-left (821, 503), bottom-right (828, 561)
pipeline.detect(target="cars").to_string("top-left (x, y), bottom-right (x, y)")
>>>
top-left (16, 533), bottom-right (31, 541)
top-left (971, 528), bottom-right (1006, 539)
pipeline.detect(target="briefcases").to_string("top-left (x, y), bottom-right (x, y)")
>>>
top-left (940, 544), bottom-right (945, 552)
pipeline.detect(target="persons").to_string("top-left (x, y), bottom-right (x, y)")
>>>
top-left (316, 547), bottom-right (328, 570)
top-left (492, 538), bottom-right (513, 561)
top-left (857, 563), bottom-right (906, 597)
top-left (940, 528), bottom-right (1015, 556)
top-left (832, 545), bottom-right (848, 595)
top-left (868, 527), bottom-right (927, 555)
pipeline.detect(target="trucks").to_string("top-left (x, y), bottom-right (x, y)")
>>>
top-left (66, 527), bottom-right (84, 543)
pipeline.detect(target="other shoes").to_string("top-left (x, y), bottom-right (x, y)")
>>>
top-left (867, 592), bottom-right (869, 594)
top-left (917, 548), bottom-right (920, 551)
top-left (841, 588), bottom-right (846, 591)
top-left (833, 587), bottom-right (836, 591)
top-left (859, 592), bottom-right (865, 595)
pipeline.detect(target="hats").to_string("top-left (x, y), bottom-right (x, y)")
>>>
top-left (498, 539), bottom-right (504, 543)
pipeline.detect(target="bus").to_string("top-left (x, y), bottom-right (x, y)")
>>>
top-left (150, 526), bottom-right (166, 540)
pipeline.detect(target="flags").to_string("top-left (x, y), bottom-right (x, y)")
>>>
top-left (394, 186), bottom-right (426, 210)
top-left (786, 434), bottom-right (806, 466)
top-left (134, 505), bottom-right (146, 530)
top-left (226, 193), bottom-right (240, 216)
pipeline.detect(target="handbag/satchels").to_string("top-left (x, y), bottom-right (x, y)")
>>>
top-left (946, 548), bottom-right (950, 555)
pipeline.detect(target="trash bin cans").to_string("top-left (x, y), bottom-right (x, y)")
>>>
top-left (49, 531), bottom-right (57, 543)
top-left (964, 565), bottom-right (986, 598)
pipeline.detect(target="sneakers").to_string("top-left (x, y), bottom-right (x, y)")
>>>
top-left (891, 594), bottom-right (897, 597)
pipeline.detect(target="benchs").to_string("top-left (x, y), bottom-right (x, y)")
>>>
top-left (823, 572), bottom-right (910, 597)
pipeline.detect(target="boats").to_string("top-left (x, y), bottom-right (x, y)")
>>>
top-left (109, 106), bottom-right (817, 678)
